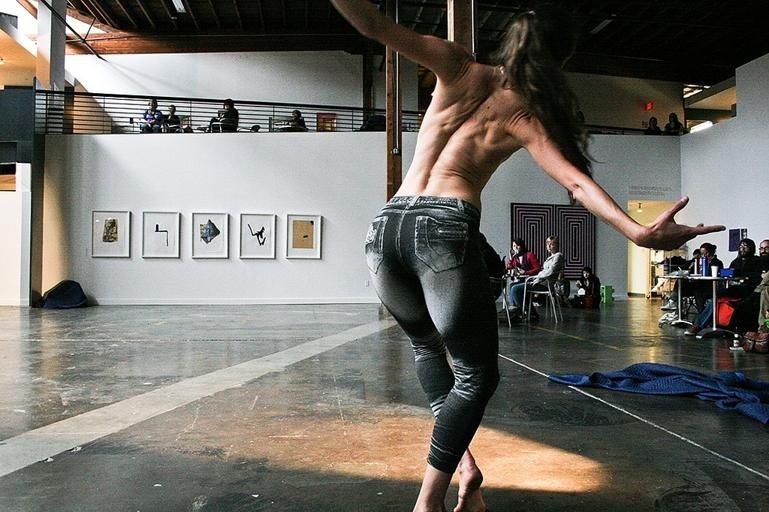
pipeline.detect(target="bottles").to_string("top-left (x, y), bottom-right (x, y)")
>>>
top-left (733, 326), bottom-right (740, 347)
top-left (693, 258), bottom-right (698, 274)
top-left (702, 256), bottom-right (708, 276)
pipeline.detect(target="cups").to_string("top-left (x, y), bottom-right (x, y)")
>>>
top-left (710, 265), bottom-right (718, 278)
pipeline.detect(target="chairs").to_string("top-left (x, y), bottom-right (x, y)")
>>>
top-left (497, 266), bottom-right (769, 348)
top-left (127, 113), bottom-right (308, 133)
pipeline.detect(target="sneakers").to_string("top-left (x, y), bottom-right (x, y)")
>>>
top-left (684, 325), bottom-right (701, 336)
top-left (660, 302), bottom-right (678, 311)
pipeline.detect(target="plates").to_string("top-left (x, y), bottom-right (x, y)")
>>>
top-left (689, 274), bottom-right (701, 277)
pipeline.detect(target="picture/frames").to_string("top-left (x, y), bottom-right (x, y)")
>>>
top-left (91, 209), bottom-right (133, 261)
top-left (238, 212), bottom-right (277, 260)
top-left (285, 212), bottom-right (322, 260)
top-left (191, 212), bottom-right (230, 260)
top-left (141, 210), bottom-right (181, 260)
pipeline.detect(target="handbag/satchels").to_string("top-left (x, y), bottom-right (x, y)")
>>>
top-left (744, 332), bottom-right (768, 353)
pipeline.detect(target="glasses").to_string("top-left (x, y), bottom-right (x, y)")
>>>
top-left (738, 245), bottom-right (748, 249)
top-left (759, 247), bottom-right (769, 250)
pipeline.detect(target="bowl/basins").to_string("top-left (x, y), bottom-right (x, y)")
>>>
top-left (678, 269), bottom-right (690, 277)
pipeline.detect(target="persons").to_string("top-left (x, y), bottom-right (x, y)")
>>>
top-left (163, 106), bottom-right (179, 132)
top-left (330, 0), bottom-right (726, 512)
top-left (288, 110), bottom-right (306, 131)
top-left (207, 99), bottom-right (239, 133)
top-left (665, 113), bottom-right (685, 135)
top-left (139, 99), bottom-right (164, 132)
top-left (476, 228), bottom-right (600, 319)
top-left (660, 238), bottom-right (769, 337)
top-left (645, 117), bottom-right (663, 135)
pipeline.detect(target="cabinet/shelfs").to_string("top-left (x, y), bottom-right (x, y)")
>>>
top-left (647, 244), bottom-right (689, 300)
top-left (315, 112), bottom-right (337, 132)
